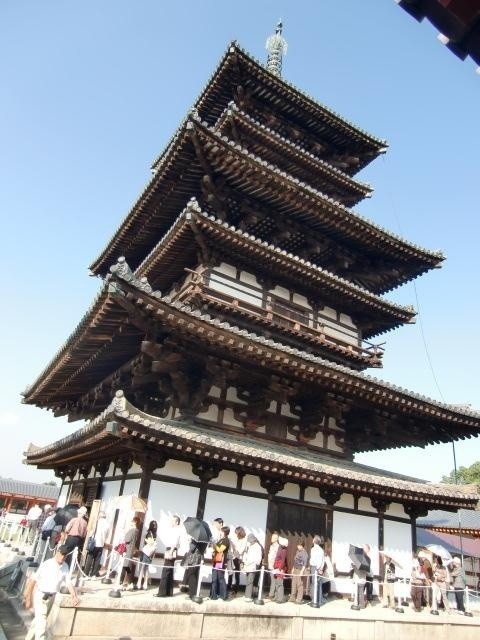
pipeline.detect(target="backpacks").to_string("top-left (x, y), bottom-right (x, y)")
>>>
top-left (141, 531), bottom-right (160, 560)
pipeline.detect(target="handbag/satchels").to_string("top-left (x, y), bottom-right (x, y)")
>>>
top-left (114, 544), bottom-right (127, 554)
top-left (86, 537), bottom-right (96, 552)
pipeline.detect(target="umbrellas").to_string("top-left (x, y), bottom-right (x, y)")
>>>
top-left (111, 493), bottom-right (149, 526)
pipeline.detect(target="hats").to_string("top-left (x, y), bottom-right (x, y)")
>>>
top-left (247, 533), bottom-right (256, 542)
top-left (76, 506), bottom-right (88, 516)
top-left (59, 544), bottom-right (70, 554)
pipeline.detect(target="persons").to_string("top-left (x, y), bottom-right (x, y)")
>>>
top-left (381, 557), bottom-right (395, 608)
top-left (410, 554), bottom-right (466, 615)
top-left (186, 518), bottom-right (263, 602)
top-left (83, 511), bottom-right (109, 577)
top-left (154, 516), bottom-right (182, 597)
top-left (20, 516), bottom-right (27, 535)
top-left (28, 502), bottom-right (88, 566)
top-left (118, 517), bottom-right (158, 591)
top-left (265, 531), bottom-right (332, 608)
top-left (1, 506), bottom-right (7, 516)
top-left (21, 542), bottom-right (81, 640)
